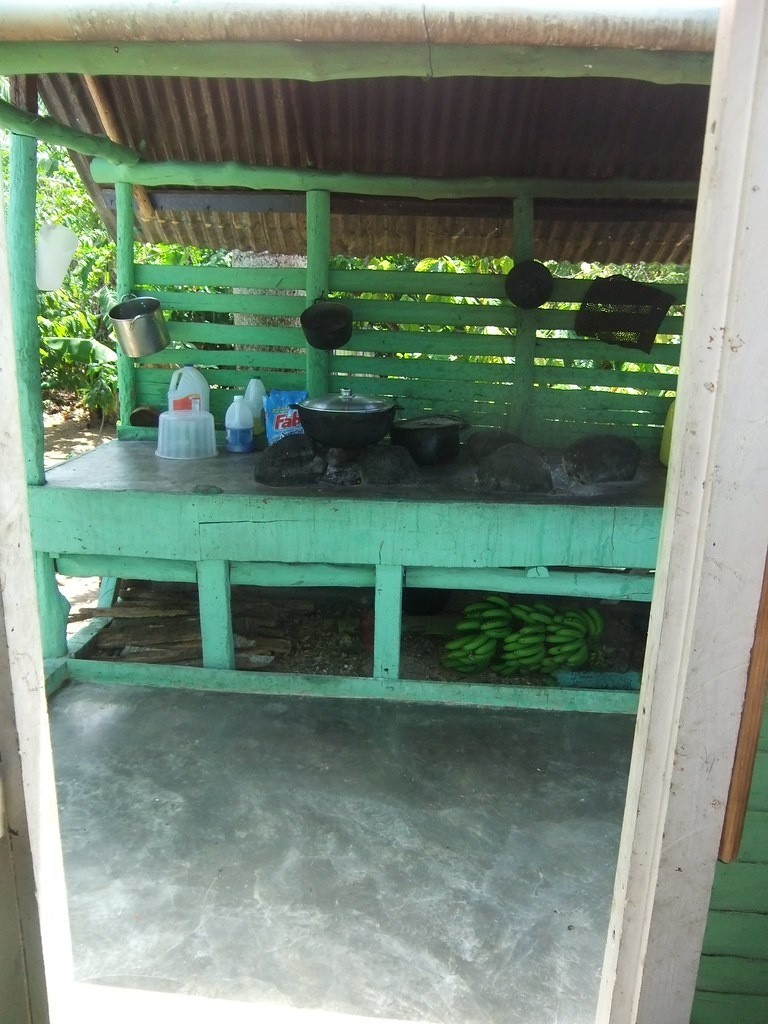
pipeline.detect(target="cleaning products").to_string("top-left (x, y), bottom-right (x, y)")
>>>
top-left (261, 387), bottom-right (309, 447)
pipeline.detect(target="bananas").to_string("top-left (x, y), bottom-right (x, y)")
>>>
top-left (440, 594), bottom-right (608, 678)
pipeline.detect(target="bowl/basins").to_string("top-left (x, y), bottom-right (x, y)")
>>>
top-left (130, 406), bottom-right (159, 428)
top-left (155, 409), bottom-right (220, 460)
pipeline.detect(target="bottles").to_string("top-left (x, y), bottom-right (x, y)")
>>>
top-left (243, 375), bottom-right (268, 451)
top-left (660, 398), bottom-right (675, 467)
top-left (225, 395), bottom-right (254, 452)
top-left (166, 362), bottom-right (210, 412)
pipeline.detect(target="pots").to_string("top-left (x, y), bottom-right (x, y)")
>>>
top-left (300, 297), bottom-right (352, 351)
top-left (389, 413), bottom-right (470, 464)
top-left (108, 292), bottom-right (170, 358)
top-left (288, 388), bottom-right (405, 448)
